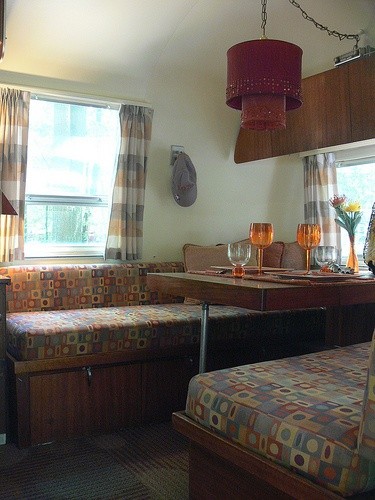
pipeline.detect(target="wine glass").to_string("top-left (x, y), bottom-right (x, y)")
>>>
top-left (249, 223), bottom-right (274, 276)
top-left (314, 245), bottom-right (337, 273)
top-left (228, 244), bottom-right (251, 278)
top-left (296, 224), bottom-right (321, 275)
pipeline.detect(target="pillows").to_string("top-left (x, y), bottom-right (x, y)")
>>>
top-left (262, 241), bottom-right (284, 267)
top-left (281, 242), bottom-right (307, 271)
top-left (183, 240), bottom-right (262, 304)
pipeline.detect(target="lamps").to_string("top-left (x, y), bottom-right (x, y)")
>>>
top-left (226, 29), bottom-right (304, 132)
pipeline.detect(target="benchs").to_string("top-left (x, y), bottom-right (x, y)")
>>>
top-left (0, 262), bottom-right (326, 448)
top-left (173, 318), bottom-right (375, 500)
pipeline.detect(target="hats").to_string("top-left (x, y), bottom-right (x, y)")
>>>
top-left (170, 152), bottom-right (197, 207)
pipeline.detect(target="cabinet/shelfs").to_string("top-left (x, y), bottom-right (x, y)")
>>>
top-left (232, 56), bottom-right (375, 164)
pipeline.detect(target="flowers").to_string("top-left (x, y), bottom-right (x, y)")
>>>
top-left (327, 193), bottom-right (362, 234)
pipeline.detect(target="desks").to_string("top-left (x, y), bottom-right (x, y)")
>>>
top-left (146, 270), bottom-right (375, 376)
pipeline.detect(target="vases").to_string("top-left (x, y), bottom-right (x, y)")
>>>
top-left (346, 234), bottom-right (359, 273)
